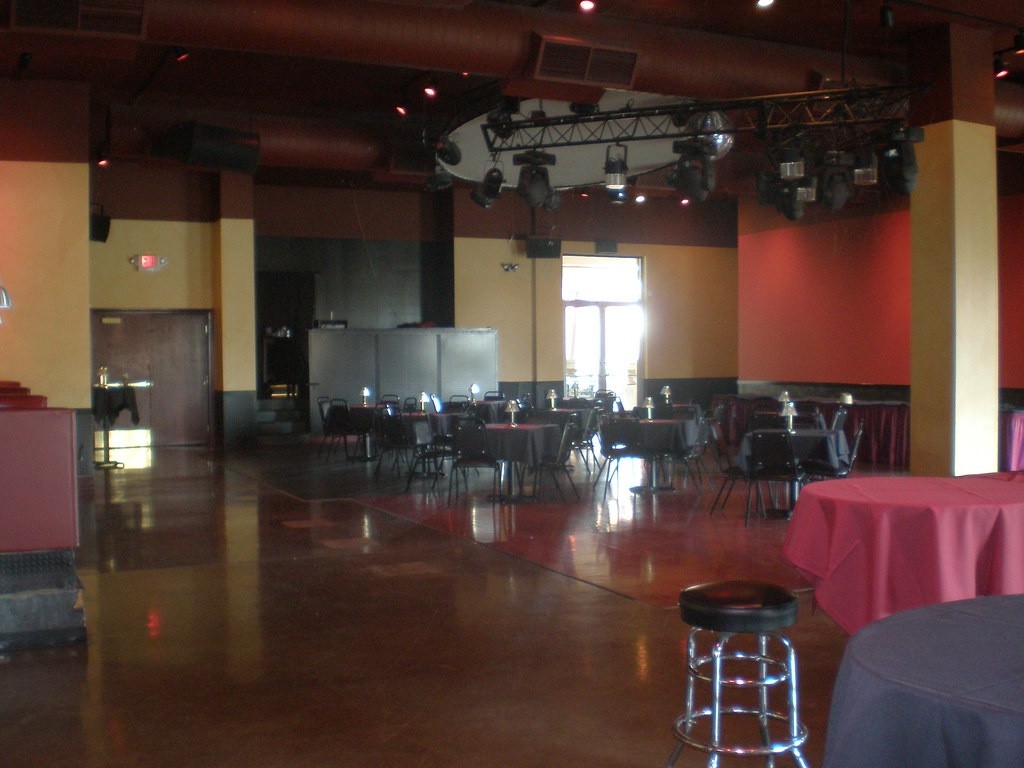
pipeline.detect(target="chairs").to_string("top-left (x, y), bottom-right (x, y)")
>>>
top-left (315, 383), bottom-right (866, 526)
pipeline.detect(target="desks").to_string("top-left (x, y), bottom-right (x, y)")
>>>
top-left (387, 412), bottom-right (462, 480)
top-left (92, 384), bottom-right (140, 469)
top-left (750, 412), bottom-right (827, 432)
top-left (461, 400), bottom-right (512, 424)
top-left (735, 428), bottom-right (850, 518)
top-left (323, 403), bottom-right (391, 462)
top-left (708, 393), bottom-right (775, 457)
top-left (524, 408), bottom-right (582, 472)
top-left (781, 476), bottom-right (1024, 638)
top-left (457, 423), bottom-right (562, 504)
top-left (822, 592), bottom-right (1024, 768)
top-left (794, 397), bottom-right (911, 469)
top-left (632, 405), bottom-right (703, 425)
top-left (559, 400), bottom-right (602, 409)
top-left (629, 420), bottom-right (698, 494)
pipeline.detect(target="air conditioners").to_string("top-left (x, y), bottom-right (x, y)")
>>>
top-left (525, 30), bottom-right (639, 90)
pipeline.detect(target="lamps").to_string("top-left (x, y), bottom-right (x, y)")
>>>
top-left (396, 99), bottom-right (414, 115)
top-left (781, 403), bottom-right (797, 434)
top-left (542, 190), bottom-right (558, 213)
top-left (993, 55), bottom-right (1008, 78)
top-left (872, 123), bottom-right (924, 195)
top-left (425, 74), bottom-right (439, 97)
top-left (1013, 33), bottom-right (1024, 54)
top-left (359, 387), bottom-right (370, 405)
top-left (880, 6), bottom-right (894, 28)
top-left (436, 138), bottom-right (461, 166)
top-left (672, 135), bottom-right (719, 203)
top-left (603, 141), bottom-right (629, 190)
top-left (503, 400), bottom-right (520, 427)
top-left (417, 391), bottom-right (430, 413)
top-left (757, 151), bottom-right (817, 221)
top-left (640, 396), bottom-right (656, 423)
top-left (546, 388), bottom-right (558, 411)
top-left (96, 142), bottom-right (110, 166)
top-left (512, 148), bottom-right (557, 208)
top-left (569, 102), bottom-right (599, 114)
top-left (470, 153), bottom-right (506, 209)
top-left (660, 385), bottom-right (672, 403)
top-left (778, 391), bottom-right (791, 407)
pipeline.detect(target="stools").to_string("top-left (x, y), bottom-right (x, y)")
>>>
top-left (665, 581), bottom-right (811, 768)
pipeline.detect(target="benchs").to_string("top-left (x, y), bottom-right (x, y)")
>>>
top-left (0, 380), bottom-right (90, 665)
